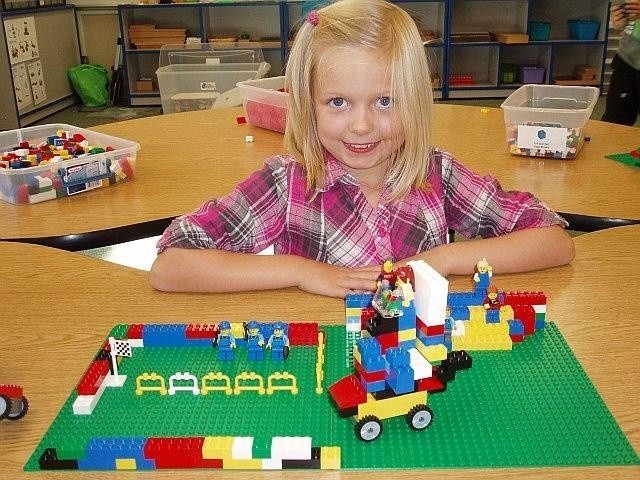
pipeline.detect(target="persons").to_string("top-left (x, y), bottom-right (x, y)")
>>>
top-left (473, 259), bottom-right (503, 322)
top-left (445, 306), bottom-right (455, 332)
top-left (216, 321), bottom-right (237, 360)
top-left (246, 321), bottom-right (264, 360)
top-left (267, 322), bottom-right (290, 361)
top-left (150, 1), bottom-right (574, 299)
top-left (376, 260), bottom-right (415, 307)
top-left (600, 1), bottom-right (640, 128)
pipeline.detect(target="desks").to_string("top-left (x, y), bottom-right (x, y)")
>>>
top-left (0, 105), bottom-right (640, 478)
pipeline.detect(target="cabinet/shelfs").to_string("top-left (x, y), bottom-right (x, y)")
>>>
top-left (285, 3), bottom-right (450, 106)
top-left (446, 2), bottom-right (613, 105)
top-left (118, 4), bottom-right (284, 105)
